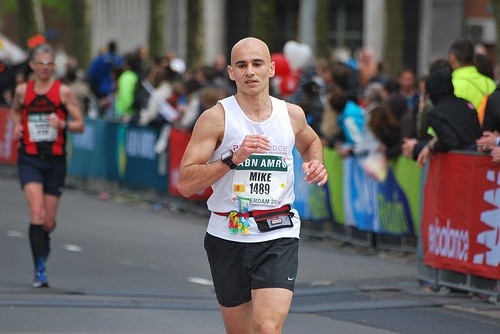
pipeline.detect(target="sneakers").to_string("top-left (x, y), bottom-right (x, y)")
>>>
top-left (34, 266), bottom-right (48, 287)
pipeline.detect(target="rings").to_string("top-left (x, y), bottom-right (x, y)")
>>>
top-left (254, 142), bottom-right (257, 148)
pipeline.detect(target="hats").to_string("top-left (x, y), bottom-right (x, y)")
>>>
top-left (426, 69), bottom-right (454, 96)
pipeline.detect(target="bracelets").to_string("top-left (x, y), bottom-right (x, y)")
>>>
top-left (63, 121), bottom-right (68, 129)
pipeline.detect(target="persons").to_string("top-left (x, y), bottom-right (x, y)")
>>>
top-left (178, 38), bottom-right (329, 334)
top-left (0, 33), bottom-right (500, 302)
top-left (12, 44), bottom-right (85, 287)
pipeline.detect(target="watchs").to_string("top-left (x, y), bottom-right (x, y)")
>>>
top-left (221, 150), bottom-right (238, 169)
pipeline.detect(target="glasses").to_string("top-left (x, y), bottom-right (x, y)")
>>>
top-left (33, 63), bottom-right (56, 68)
